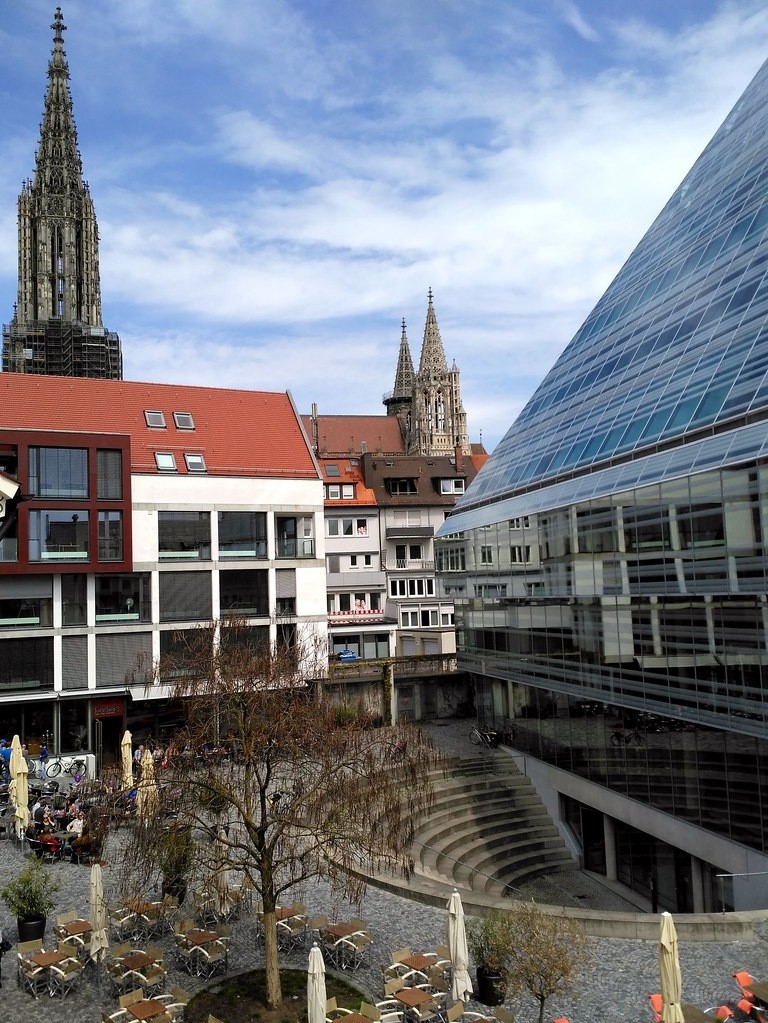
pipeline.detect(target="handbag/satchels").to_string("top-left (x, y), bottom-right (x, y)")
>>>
top-left (4, 761), bottom-right (9, 768)
top-left (44, 758), bottom-right (49, 764)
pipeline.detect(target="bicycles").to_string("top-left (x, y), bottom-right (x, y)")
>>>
top-left (45, 756), bottom-right (87, 779)
top-left (611, 727), bottom-right (647, 746)
top-left (470, 721), bottom-right (498, 747)
top-left (0, 759), bottom-right (42, 782)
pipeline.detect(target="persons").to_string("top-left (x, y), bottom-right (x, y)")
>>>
top-left (0, 739), bottom-right (28, 784)
top-left (41, 830), bottom-right (61, 862)
top-left (133, 744), bottom-right (145, 773)
top-left (161, 742), bottom-right (180, 769)
top-left (39, 812), bottom-right (56, 830)
top-left (37, 742), bottom-right (49, 781)
top-left (152, 746), bottom-right (165, 756)
top-left (34, 800), bottom-right (47, 829)
top-left (67, 811), bottom-right (87, 844)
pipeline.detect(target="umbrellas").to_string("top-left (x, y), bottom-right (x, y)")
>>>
top-left (120, 730), bottom-right (133, 792)
top-left (135, 749), bottom-right (160, 829)
top-left (14, 756), bottom-right (30, 851)
top-left (88, 864), bottom-right (109, 987)
top-left (307, 942), bottom-right (327, 1023)
top-left (446, 888), bottom-right (473, 1023)
top-left (213, 829), bottom-right (232, 926)
top-left (658, 912), bottom-right (685, 1023)
top-left (8, 735), bottom-right (23, 807)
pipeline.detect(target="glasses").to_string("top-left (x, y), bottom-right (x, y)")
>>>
top-left (78, 815), bottom-right (82, 816)
top-left (43, 817), bottom-right (48, 819)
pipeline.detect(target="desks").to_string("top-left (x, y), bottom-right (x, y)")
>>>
top-left (186, 930), bottom-right (219, 973)
top-left (63, 921), bottom-right (93, 957)
top-left (51, 811), bottom-right (67, 831)
top-left (62, 545), bottom-right (80, 559)
top-left (743, 981), bottom-right (768, 1009)
top-left (52, 830), bottom-right (79, 864)
top-left (274, 905), bottom-right (298, 947)
top-left (394, 986), bottom-right (432, 1021)
top-left (399, 953), bottom-right (436, 986)
top-left (47, 545), bottom-right (62, 561)
top-left (127, 999), bottom-right (167, 1021)
top-left (29, 950), bottom-right (67, 994)
top-left (680, 1004), bottom-right (717, 1023)
top-left (119, 953), bottom-right (156, 990)
top-left (332, 1012), bottom-right (372, 1023)
top-left (324, 923), bottom-right (358, 963)
top-left (127, 901), bottom-right (160, 938)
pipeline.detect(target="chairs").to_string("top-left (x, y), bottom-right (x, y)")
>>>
top-left (175, 882), bottom-right (252, 980)
top-left (53, 941), bottom-right (78, 962)
top-left (144, 944), bottom-right (163, 974)
top-left (138, 906), bottom-right (166, 942)
top-left (0, 731), bottom-right (290, 870)
top-left (53, 926), bottom-right (69, 949)
top-left (106, 963), bottom-right (132, 998)
top-left (255, 896), bottom-right (768, 1023)
top-left (17, 953), bottom-right (48, 1000)
top-left (133, 960), bottom-right (167, 994)
top-left (100, 1011), bottom-right (178, 1023)
top-left (17, 939), bottom-right (45, 988)
top-left (56, 909), bottom-right (77, 928)
top-left (161, 894), bottom-right (180, 931)
top-left (118, 988), bottom-right (150, 1021)
top-left (111, 941), bottom-right (140, 972)
top-left (76, 936), bottom-right (93, 963)
top-left (107, 907), bottom-right (138, 942)
top-left (152, 985), bottom-right (188, 1019)
top-left (50, 957), bottom-right (86, 1001)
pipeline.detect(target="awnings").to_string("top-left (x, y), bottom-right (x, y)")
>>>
top-left (130, 676), bottom-right (308, 702)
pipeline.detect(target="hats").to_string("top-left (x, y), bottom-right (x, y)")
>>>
top-left (0, 739), bottom-right (6, 743)
